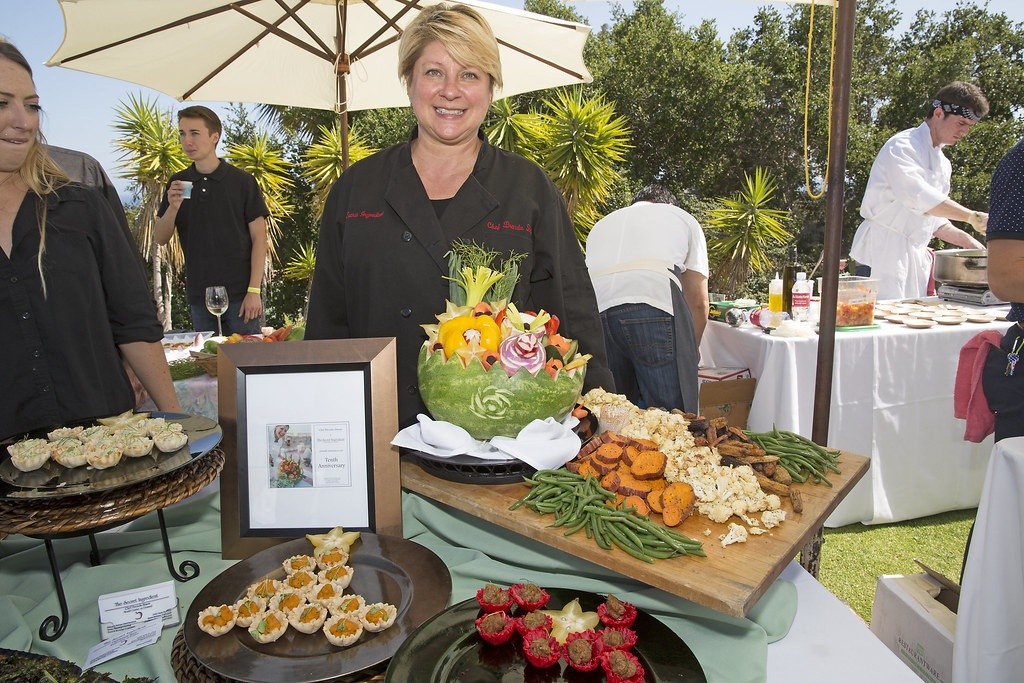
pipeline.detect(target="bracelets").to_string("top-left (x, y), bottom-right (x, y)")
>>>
top-left (248, 287), bottom-right (260, 294)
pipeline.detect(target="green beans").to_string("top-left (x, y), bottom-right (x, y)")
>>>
top-left (509, 468), bottom-right (708, 565)
top-left (742, 422), bottom-right (842, 488)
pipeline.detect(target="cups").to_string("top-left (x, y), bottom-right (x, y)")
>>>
top-left (179, 181), bottom-right (193, 199)
top-left (261, 327), bottom-right (273, 336)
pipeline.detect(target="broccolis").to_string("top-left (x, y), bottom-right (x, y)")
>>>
top-left (581, 387), bottom-right (786, 548)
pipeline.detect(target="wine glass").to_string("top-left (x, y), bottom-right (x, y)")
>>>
top-left (205, 286), bottom-right (229, 341)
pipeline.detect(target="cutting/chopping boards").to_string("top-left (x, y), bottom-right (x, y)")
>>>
top-left (400, 410), bottom-right (872, 619)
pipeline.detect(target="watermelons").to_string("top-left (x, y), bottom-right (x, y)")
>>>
top-left (417, 341), bottom-right (586, 440)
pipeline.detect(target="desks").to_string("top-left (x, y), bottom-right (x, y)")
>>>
top-left (398, 447), bottom-right (874, 618)
top-left (697, 295), bottom-right (1018, 527)
top-left (950, 435), bottom-right (1024, 683)
top-left (0, 473), bottom-right (925, 683)
top-left (135, 333), bottom-right (265, 423)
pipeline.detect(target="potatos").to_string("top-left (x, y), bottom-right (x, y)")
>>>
top-left (565, 430), bottom-right (695, 526)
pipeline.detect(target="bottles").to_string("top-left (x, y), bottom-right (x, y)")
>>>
top-left (782, 245), bottom-right (801, 319)
top-left (791, 272), bottom-right (811, 322)
top-left (744, 308), bottom-right (790, 329)
top-left (769, 271), bottom-right (783, 312)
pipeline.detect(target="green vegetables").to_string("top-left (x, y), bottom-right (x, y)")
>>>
top-left (442, 237), bottom-right (529, 309)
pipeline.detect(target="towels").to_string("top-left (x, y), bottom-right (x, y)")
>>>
top-left (490, 415), bottom-right (584, 473)
top-left (953, 330), bottom-right (1004, 445)
top-left (388, 413), bottom-right (518, 459)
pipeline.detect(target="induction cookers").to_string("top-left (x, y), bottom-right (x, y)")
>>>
top-left (936, 283), bottom-right (1012, 305)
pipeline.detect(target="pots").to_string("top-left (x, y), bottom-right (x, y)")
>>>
top-left (931, 248), bottom-right (988, 287)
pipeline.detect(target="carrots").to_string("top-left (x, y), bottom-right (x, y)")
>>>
top-left (474, 302), bottom-right (570, 378)
top-left (268, 325), bottom-right (294, 343)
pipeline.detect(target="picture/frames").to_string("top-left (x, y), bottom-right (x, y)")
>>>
top-left (215, 336), bottom-right (402, 561)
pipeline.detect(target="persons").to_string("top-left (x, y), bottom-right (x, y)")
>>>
top-left (959, 136), bottom-right (1024, 587)
top-left (0, 33), bottom-right (184, 444)
top-left (302, 2), bottom-right (617, 455)
top-left (154, 106), bottom-right (270, 337)
top-left (585, 184), bottom-right (709, 417)
top-left (847, 83), bottom-right (989, 301)
top-left (268, 424), bottom-right (289, 466)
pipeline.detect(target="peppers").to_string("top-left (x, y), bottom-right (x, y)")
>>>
top-left (438, 314), bottom-right (501, 359)
top-left (201, 333), bottom-right (243, 354)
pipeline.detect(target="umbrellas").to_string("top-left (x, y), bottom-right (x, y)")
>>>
top-left (43, 1), bottom-right (592, 170)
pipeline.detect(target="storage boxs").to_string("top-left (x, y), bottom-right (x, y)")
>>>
top-left (835, 275), bottom-right (881, 327)
top-left (697, 366), bottom-right (752, 392)
top-left (163, 331), bottom-right (215, 341)
top-left (869, 557), bottom-right (962, 683)
top-left (698, 378), bottom-right (757, 430)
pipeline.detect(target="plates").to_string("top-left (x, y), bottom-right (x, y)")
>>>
top-left (403, 402), bottom-right (599, 484)
top-left (874, 299), bottom-right (1010, 328)
top-left (163, 330), bottom-right (215, 362)
top-left (384, 586), bottom-right (707, 683)
top-left (184, 531), bottom-right (453, 683)
top-left (0, 411), bottom-right (224, 496)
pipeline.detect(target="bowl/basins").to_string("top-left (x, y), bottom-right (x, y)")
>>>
top-left (816, 275), bottom-right (881, 327)
